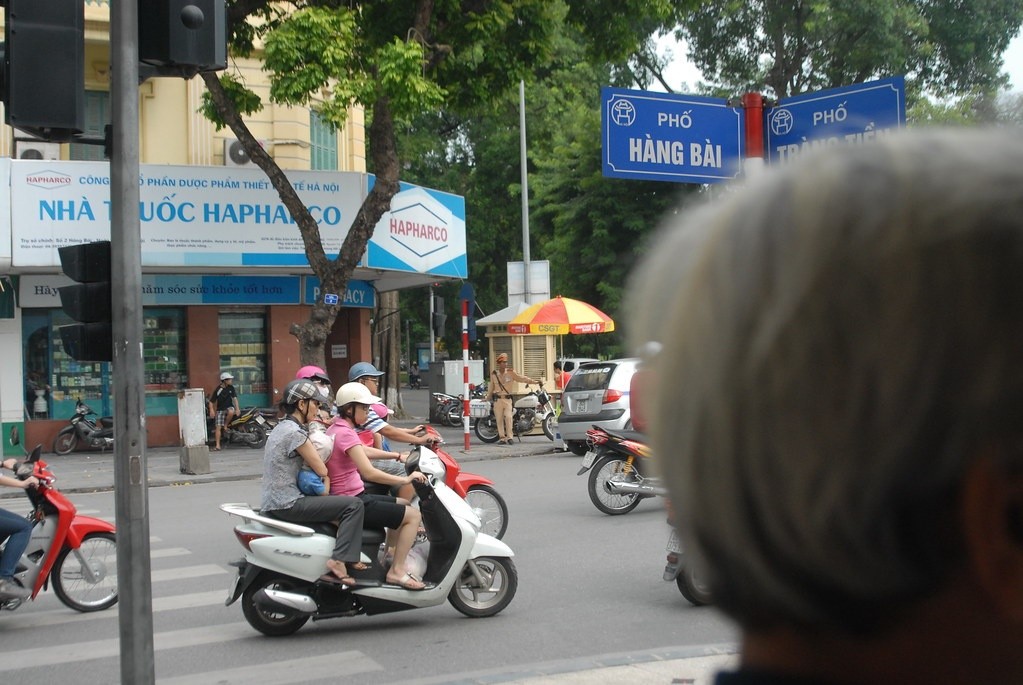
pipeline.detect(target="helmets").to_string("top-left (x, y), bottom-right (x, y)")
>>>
top-left (639, 341), bottom-right (665, 362)
top-left (220, 372), bottom-right (234, 381)
top-left (282, 379), bottom-right (327, 403)
top-left (348, 362), bottom-right (386, 382)
top-left (335, 382), bottom-right (383, 408)
top-left (296, 365), bottom-right (332, 385)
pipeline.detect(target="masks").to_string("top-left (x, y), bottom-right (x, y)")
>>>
top-left (318, 387), bottom-right (329, 398)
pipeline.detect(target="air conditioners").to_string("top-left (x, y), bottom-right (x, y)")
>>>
top-left (16, 141), bottom-right (60, 161)
top-left (223, 138), bottom-right (269, 170)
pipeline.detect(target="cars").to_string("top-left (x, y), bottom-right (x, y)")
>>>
top-left (559, 359), bottom-right (598, 376)
top-left (557, 356), bottom-right (640, 455)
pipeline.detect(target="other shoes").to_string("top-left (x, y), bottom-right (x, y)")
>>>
top-left (508, 439), bottom-right (514, 445)
top-left (0, 580), bottom-right (33, 599)
top-left (498, 439), bottom-right (506, 445)
top-left (210, 447), bottom-right (221, 451)
top-left (224, 428), bottom-right (229, 433)
top-left (348, 562), bottom-right (368, 570)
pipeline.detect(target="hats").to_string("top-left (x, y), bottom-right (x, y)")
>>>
top-left (413, 361), bottom-right (416, 364)
top-left (497, 353), bottom-right (508, 363)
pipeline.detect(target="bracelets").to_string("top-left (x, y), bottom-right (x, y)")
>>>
top-left (396, 453), bottom-right (401, 462)
top-left (1, 461), bottom-right (4, 468)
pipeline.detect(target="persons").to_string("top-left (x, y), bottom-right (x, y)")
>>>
top-left (630, 342), bottom-right (660, 434)
top-left (409, 361), bottom-right (420, 386)
top-left (621, 121), bottom-right (1023, 684)
top-left (480, 353), bottom-right (542, 445)
top-left (0, 459), bottom-right (39, 600)
top-left (260, 362), bottom-right (444, 590)
top-left (209, 372), bottom-right (241, 450)
top-left (552, 361), bottom-right (572, 390)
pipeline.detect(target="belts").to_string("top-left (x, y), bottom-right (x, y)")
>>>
top-left (497, 396), bottom-right (511, 398)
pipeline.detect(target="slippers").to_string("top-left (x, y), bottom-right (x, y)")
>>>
top-left (386, 573), bottom-right (427, 590)
top-left (320, 573), bottom-right (356, 585)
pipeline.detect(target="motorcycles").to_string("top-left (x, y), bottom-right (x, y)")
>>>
top-left (576, 423), bottom-right (673, 516)
top-left (244, 385), bottom-right (288, 432)
top-left (205, 394), bottom-right (267, 449)
top-left (218, 420), bottom-right (518, 636)
top-left (0, 425), bottom-right (118, 612)
top-left (431, 381), bottom-right (559, 443)
top-left (53, 396), bottom-right (114, 456)
top-left (663, 500), bottom-right (715, 605)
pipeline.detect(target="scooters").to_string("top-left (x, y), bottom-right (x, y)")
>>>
top-left (407, 374), bottom-right (422, 390)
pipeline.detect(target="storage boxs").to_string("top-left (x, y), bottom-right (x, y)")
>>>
top-left (469, 400), bottom-right (491, 418)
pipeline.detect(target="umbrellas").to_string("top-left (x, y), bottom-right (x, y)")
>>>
top-left (507, 295), bottom-right (615, 389)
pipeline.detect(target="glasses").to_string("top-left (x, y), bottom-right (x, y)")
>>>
top-left (310, 399), bottom-right (321, 405)
top-left (312, 380), bottom-right (329, 385)
top-left (352, 403), bottom-right (371, 410)
top-left (362, 377), bottom-right (380, 383)
